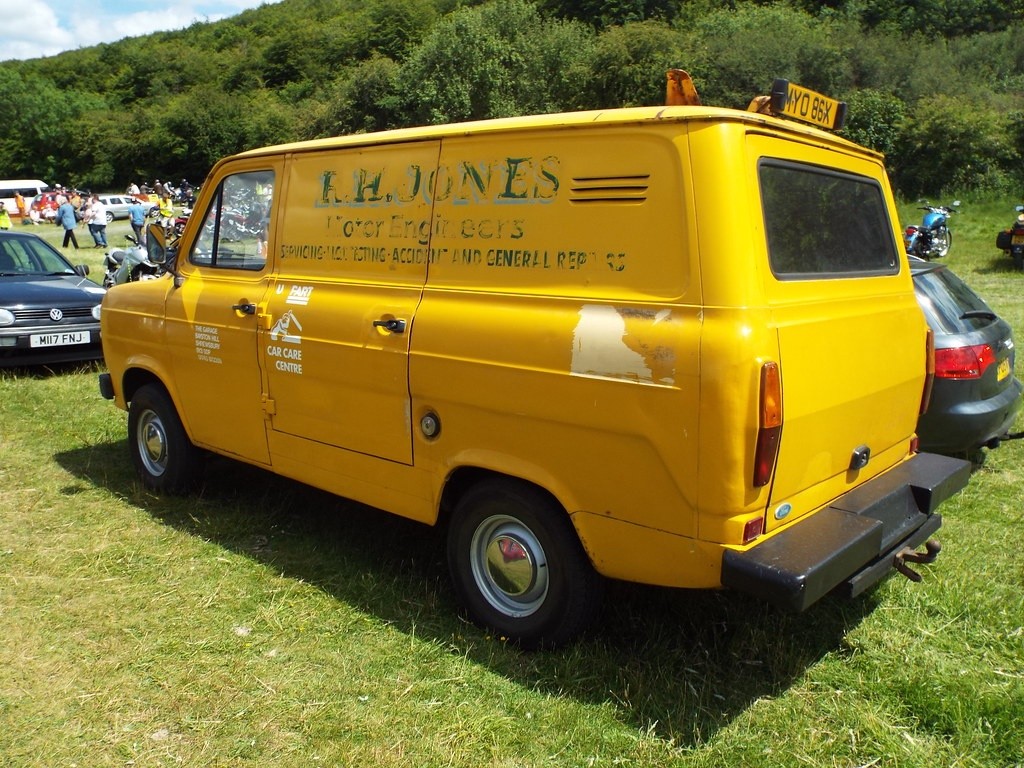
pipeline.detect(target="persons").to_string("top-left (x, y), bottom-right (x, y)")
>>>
top-left (15, 191), bottom-right (26, 222)
top-left (127, 178), bottom-right (203, 209)
top-left (128, 198), bottom-right (145, 247)
top-left (29, 206), bottom-right (43, 225)
top-left (256, 182), bottom-right (273, 204)
top-left (0, 202), bottom-right (13, 230)
top-left (46, 185), bottom-right (107, 248)
top-left (157, 193), bottom-right (175, 236)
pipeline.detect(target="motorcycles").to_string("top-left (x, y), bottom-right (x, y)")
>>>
top-left (173, 209), bottom-right (263, 242)
top-left (158, 235), bottom-right (210, 273)
top-left (997, 206), bottom-right (1024, 262)
top-left (102, 234), bottom-right (161, 291)
top-left (900, 197), bottom-right (961, 263)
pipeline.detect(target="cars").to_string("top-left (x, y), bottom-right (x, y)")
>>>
top-left (0, 226), bottom-right (111, 370)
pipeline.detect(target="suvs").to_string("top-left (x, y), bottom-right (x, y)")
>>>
top-left (903, 254), bottom-right (1024, 473)
top-left (29, 190), bottom-right (92, 223)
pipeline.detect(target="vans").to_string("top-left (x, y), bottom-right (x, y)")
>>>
top-left (88, 195), bottom-right (160, 223)
top-left (0, 180), bottom-right (50, 216)
top-left (99, 68), bottom-right (971, 650)
